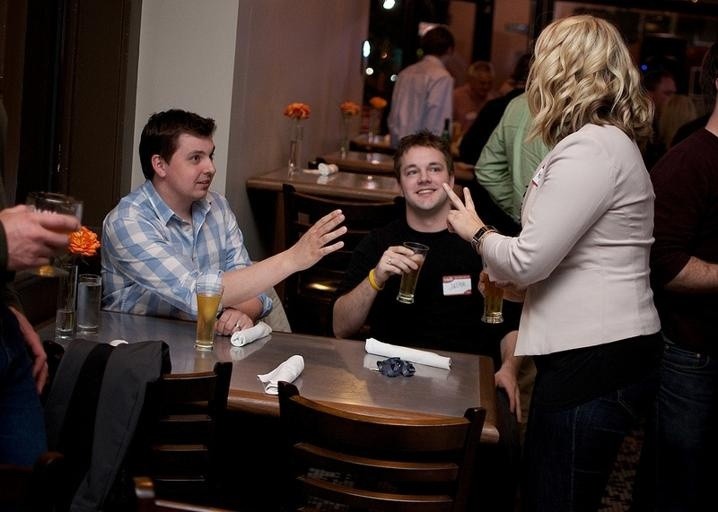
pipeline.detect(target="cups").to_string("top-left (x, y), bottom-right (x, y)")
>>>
top-left (77, 273), bottom-right (103, 333)
top-left (481, 266), bottom-right (504, 324)
top-left (397, 241), bottom-right (430, 304)
top-left (193, 282), bottom-right (225, 352)
top-left (26, 192), bottom-right (82, 278)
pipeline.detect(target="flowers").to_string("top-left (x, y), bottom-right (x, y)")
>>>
top-left (62, 226), bottom-right (102, 327)
top-left (370, 96), bottom-right (387, 133)
top-left (283, 101), bottom-right (310, 165)
top-left (340, 101), bottom-right (359, 144)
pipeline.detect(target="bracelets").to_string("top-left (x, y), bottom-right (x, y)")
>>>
top-left (368, 268), bottom-right (386, 292)
top-left (469, 225), bottom-right (500, 254)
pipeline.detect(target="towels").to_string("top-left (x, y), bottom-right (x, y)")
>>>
top-left (228, 322), bottom-right (454, 396)
top-left (319, 163), bottom-right (338, 176)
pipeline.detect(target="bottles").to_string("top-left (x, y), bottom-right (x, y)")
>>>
top-left (441, 118), bottom-right (452, 145)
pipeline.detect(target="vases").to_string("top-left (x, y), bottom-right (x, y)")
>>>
top-left (288, 122), bottom-right (304, 177)
top-left (55, 263), bottom-right (79, 342)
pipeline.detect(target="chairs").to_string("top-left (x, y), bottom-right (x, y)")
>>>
top-left (134, 476), bottom-right (236, 511)
top-left (282, 183), bottom-right (403, 333)
top-left (1, 452), bottom-right (73, 511)
top-left (44, 339), bottom-right (232, 512)
top-left (278, 382), bottom-right (486, 511)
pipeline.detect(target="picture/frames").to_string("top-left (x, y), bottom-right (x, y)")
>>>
top-left (368, 109), bottom-right (381, 135)
top-left (338, 120), bottom-right (352, 157)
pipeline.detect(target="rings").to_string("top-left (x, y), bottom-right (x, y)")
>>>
top-left (234, 324), bottom-right (242, 331)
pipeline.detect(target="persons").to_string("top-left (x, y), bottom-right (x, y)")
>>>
top-left (622, 30), bottom-right (717, 172)
top-left (386, 26), bottom-right (457, 146)
top-left (328, 128), bottom-right (526, 431)
top-left (98, 105), bottom-right (351, 335)
top-left (648, 41), bottom-right (718, 512)
top-left (438, 14), bottom-right (665, 512)
top-left (458, 54), bottom-right (533, 166)
top-left (0, 202), bottom-right (81, 512)
top-left (450, 60), bottom-right (494, 128)
